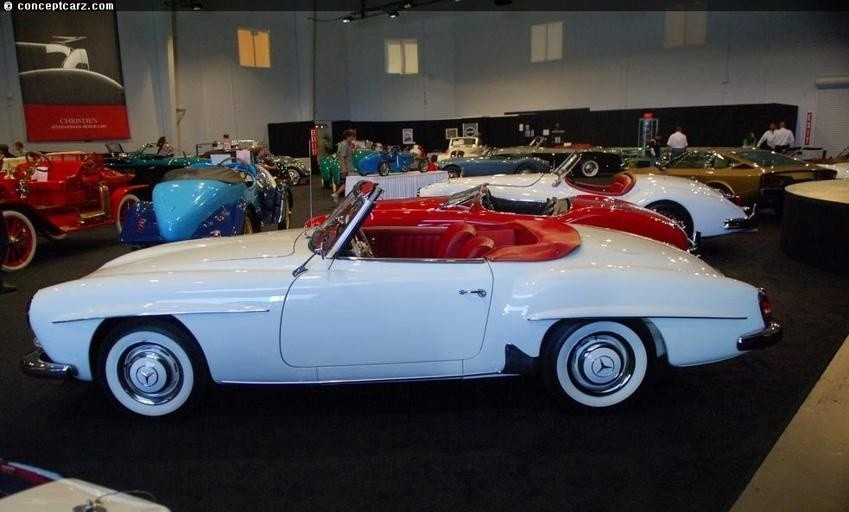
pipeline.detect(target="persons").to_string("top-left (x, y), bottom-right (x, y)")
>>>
top-left (650, 132), bottom-right (664, 166)
top-left (13, 141), bottom-right (27, 157)
top-left (667, 127), bottom-right (689, 159)
top-left (331, 129), bottom-right (357, 204)
top-left (757, 122), bottom-right (794, 152)
top-left (743, 130), bottom-right (757, 150)
top-left (253, 147), bottom-right (268, 162)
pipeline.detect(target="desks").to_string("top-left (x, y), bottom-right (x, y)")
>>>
top-left (348, 169), bottom-right (451, 205)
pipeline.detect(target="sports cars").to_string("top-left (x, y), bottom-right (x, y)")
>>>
top-left (28, 179), bottom-right (808, 411)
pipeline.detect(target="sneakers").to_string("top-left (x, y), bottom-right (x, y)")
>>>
top-left (330, 193), bottom-right (339, 204)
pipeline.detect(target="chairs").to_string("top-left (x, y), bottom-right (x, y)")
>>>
top-left (608, 173), bottom-right (633, 193)
top-left (542, 194), bottom-right (568, 217)
top-left (19, 159), bottom-right (83, 207)
top-left (436, 222), bottom-right (497, 259)
